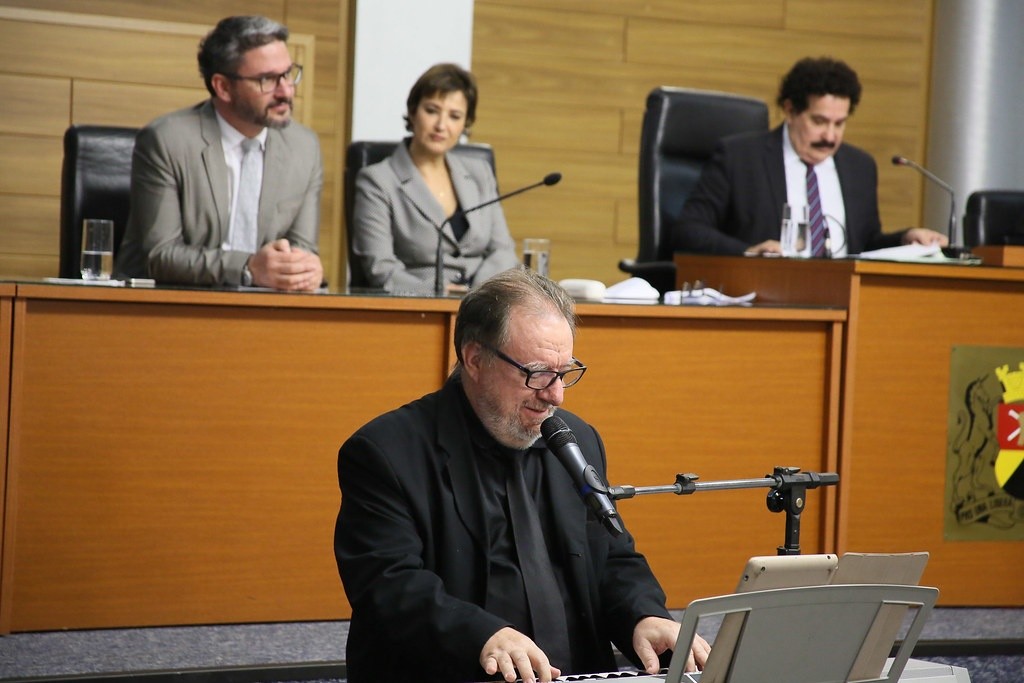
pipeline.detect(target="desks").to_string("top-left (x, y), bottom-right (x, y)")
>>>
top-left (675, 253), bottom-right (1024, 605)
top-left (1, 279), bottom-right (846, 637)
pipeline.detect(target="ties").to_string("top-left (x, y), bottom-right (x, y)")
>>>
top-left (504, 447), bottom-right (573, 675)
top-left (802, 159), bottom-right (826, 259)
top-left (231, 138), bottom-right (263, 256)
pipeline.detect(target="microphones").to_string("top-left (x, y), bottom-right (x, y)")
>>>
top-left (892, 155), bottom-right (968, 259)
top-left (540, 416), bottom-right (623, 538)
top-left (435, 172), bottom-right (562, 298)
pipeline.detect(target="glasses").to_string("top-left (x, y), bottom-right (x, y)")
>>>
top-left (221, 63), bottom-right (303, 93)
top-left (476, 340), bottom-right (587, 390)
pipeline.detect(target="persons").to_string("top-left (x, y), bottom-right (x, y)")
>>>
top-left (112, 15), bottom-right (324, 292)
top-left (677, 57), bottom-right (949, 254)
top-left (333, 267), bottom-right (712, 682)
top-left (353, 63), bottom-right (516, 294)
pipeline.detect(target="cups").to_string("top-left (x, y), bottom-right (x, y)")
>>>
top-left (780, 203), bottom-right (812, 258)
top-left (80, 219), bottom-right (113, 280)
top-left (523, 238), bottom-right (549, 278)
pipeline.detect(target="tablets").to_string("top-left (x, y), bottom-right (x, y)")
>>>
top-left (698, 553), bottom-right (838, 683)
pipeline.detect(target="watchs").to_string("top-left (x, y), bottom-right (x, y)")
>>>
top-left (241, 266), bottom-right (253, 286)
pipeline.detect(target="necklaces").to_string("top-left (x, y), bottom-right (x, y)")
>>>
top-left (437, 192), bottom-right (445, 196)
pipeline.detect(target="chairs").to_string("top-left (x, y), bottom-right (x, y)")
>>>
top-left (344, 140), bottom-right (498, 282)
top-left (962, 190), bottom-right (1024, 248)
top-left (60, 124), bottom-right (141, 278)
top-left (618, 86), bottom-right (768, 292)
